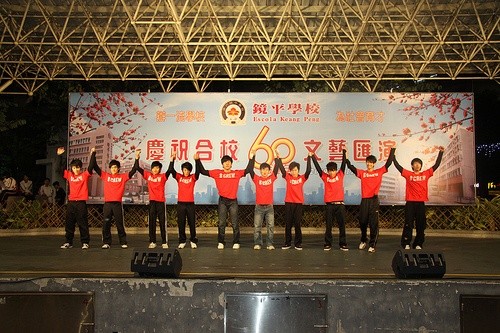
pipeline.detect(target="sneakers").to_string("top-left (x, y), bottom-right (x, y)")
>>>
top-left (102, 244), bottom-right (110, 249)
top-left (162, 243), bottom-right (168, 249)
top-left (416, 245), bottom-right (422, 250)
top-left (233, 244), bottom-right (239, 249)
top-left (368, 247), bottom-right (375, 252)
top-left (190, 242), bottom-right (197, 248)
top-left (254, 245), bottom-right (261, 250)
top-left (149, 242), bottom-right (156, 249)
top-left (178, 242), bottom-right (186, 249)
top-left (295, 245), bottom-right (303, 250)
top-left (359, 242), bottom-right (366, 250)
top-left (82, 243), bottom-right (89, 249)
top-left (282, 244), bottom-right (292, 250)
top-left (340, 246), bottom-right (349, 251)
top-left (122, 244), bottom-right (128, 248)
top-left (60, 243), bottom-right (72, 249)
top-left (267, 246), bottom-right (275, 250)
top-left (324, 246), bottom-right (331, 251)
top-left (405, 245), bottom-right (411, 250)
top-left (218, 243), bottom-right (224, 249)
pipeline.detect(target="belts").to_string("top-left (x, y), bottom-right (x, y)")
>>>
top-left (331, 202), bottom-right (344, 204)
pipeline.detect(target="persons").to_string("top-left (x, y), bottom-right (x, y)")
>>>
top-left (35, 177), bottom-right (56, 204)
top-left (18, 172), bottom-right (34, 202)
top-left (0, 172), bottom-right (19, 205)
top-left (57, 145), bottom-right (444, 253)
top-left (53, 181), bottom-right (66, 206)
